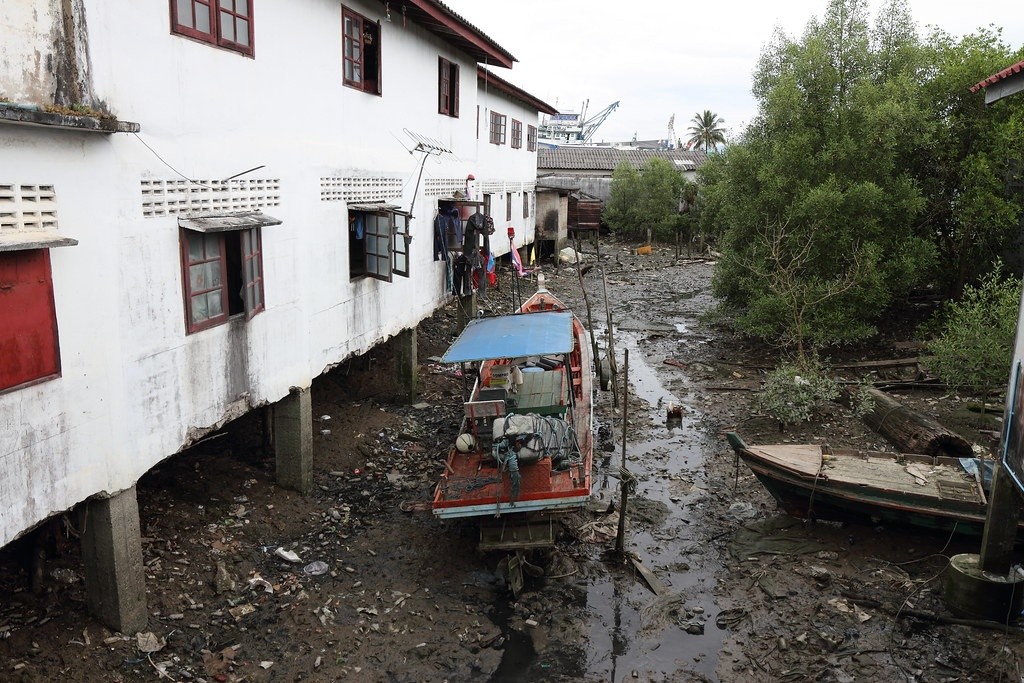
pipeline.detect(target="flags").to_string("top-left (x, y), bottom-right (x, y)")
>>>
top-left (511, 243), bottom-right (532, 278)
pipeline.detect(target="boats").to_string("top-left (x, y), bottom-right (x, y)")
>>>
top-left (431, 277), bottom-right (594, 590)
top-left (726, 430), bottom-right (994, 541)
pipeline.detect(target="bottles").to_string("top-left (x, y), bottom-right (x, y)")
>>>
top-left (511, 365), bottom-right (523, 385)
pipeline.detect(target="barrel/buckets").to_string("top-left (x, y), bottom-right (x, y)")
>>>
top-left (491, 364), bottom-right (512, 390)
